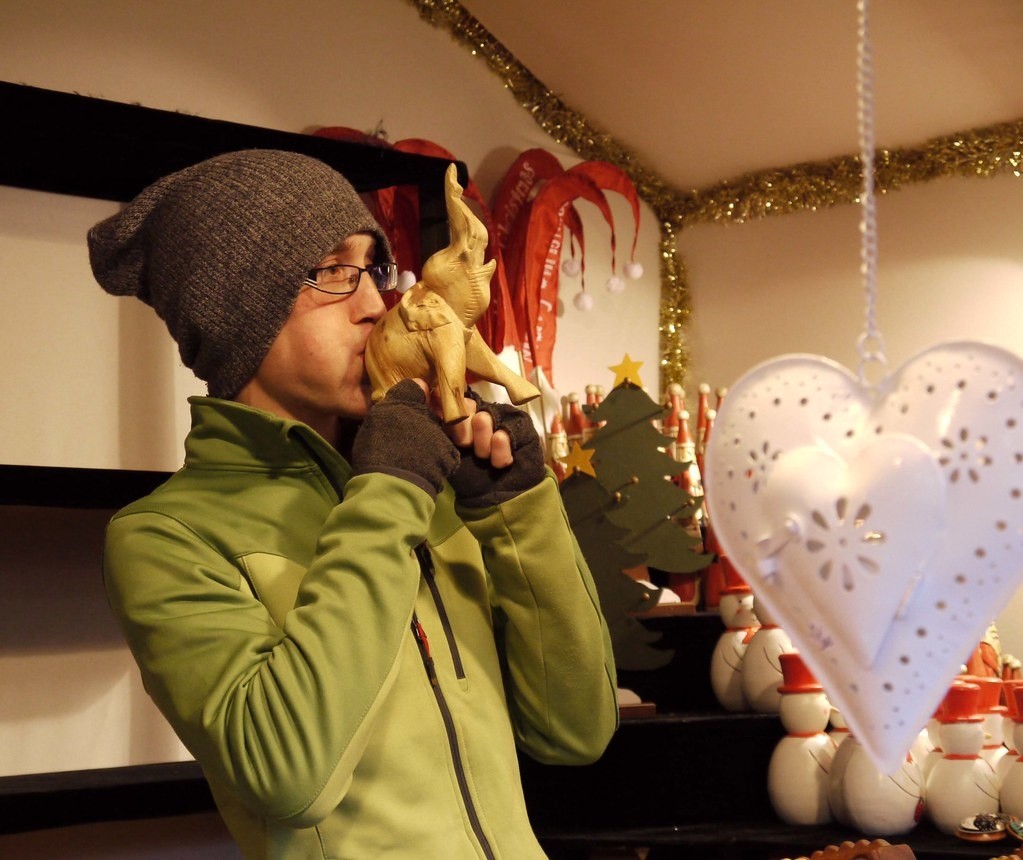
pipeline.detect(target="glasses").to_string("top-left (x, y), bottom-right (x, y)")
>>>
top-left (303, 262), bottom-right (402, 295)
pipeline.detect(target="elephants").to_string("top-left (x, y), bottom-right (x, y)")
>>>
top-left (365, 163), bottom-right (542, 424)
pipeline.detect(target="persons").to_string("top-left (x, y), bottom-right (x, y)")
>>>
top-left (83, 147), bottom-right (622, 860)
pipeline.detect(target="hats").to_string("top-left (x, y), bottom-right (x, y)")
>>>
top-left (86, 148), bottom-right (397, 401)
